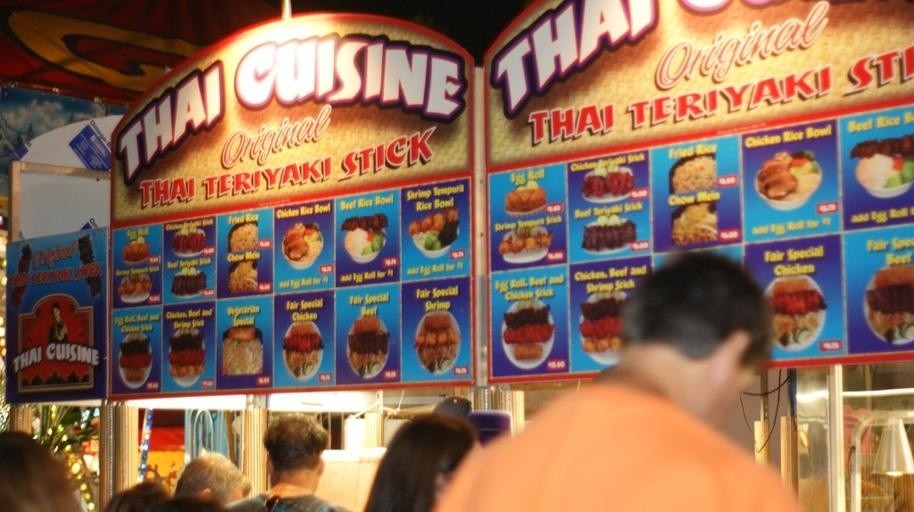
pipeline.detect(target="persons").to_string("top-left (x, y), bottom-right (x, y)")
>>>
top-left (434, 252), bottom-right (806, 512)
top-left (0, 430), bottom-right (82, 512)
top-left (363, 413), bottom-right (482, 512)
top-left (105, 453), bottom-right (252, 512)
top-left (221, 413), bottom-right (336, 512)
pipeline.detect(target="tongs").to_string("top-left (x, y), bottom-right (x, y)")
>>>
top-left (856, 155), bottom-right (893, 190)
top-left (672, 204), bottom-right (715, 245)
top-left (783, 169), bottom-right (822, 202)
top-left (673, 155), bottom-right (716, 192)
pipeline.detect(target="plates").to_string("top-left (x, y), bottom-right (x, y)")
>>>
top-left (120, 151), bottom-right (912, 388)
